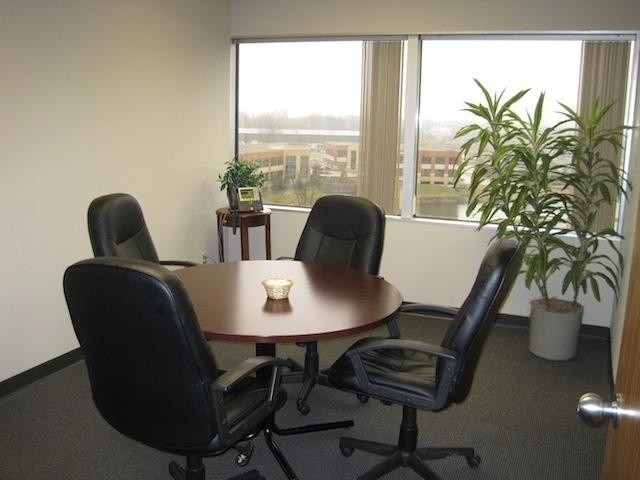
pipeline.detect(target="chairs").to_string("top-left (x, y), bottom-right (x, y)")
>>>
top-left (327, 237), bottom-right (524, 479)
top-left (87, 193), bottom-right (200, 267)
top-left (64, 256), bottom-right (298, 480)
top-left (275, 194), bottom-right (393, 415)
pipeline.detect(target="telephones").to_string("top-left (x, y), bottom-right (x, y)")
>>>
top-left (227, 183), bottom-right (263, 212)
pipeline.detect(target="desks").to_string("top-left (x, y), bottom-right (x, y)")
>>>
top-left (170, 260), bottom-right (403, 480)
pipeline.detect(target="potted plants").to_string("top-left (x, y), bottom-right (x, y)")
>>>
top-left (216, 154), bottom-right (268, 212)
top-left (447, 72), bottom-right (627, 364)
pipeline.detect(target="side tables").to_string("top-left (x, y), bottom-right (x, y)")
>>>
top-left (216, 206), bottom-right (272, 261)
